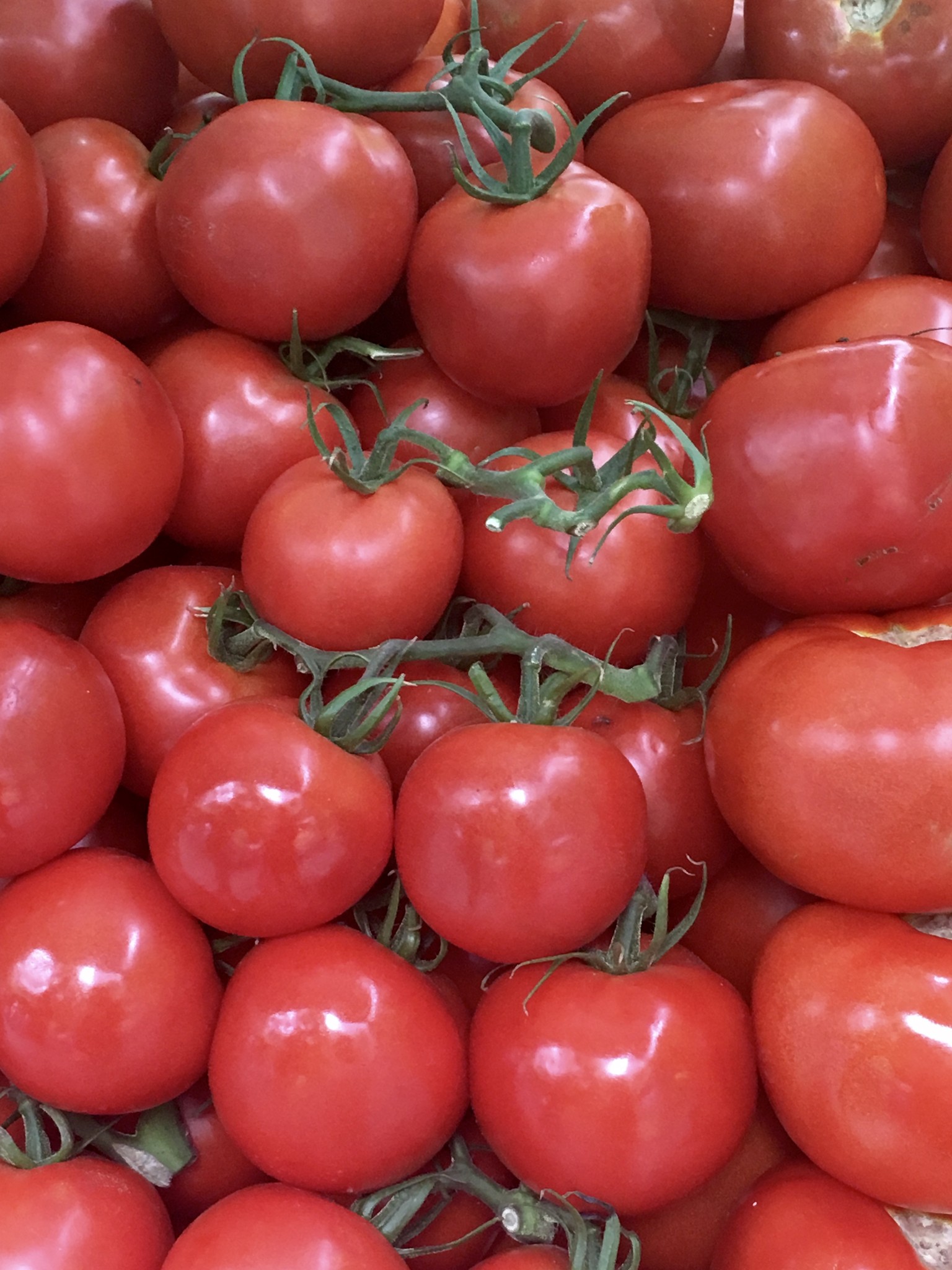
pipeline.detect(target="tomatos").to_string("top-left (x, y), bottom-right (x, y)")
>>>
top-left (0, 4), bottom-right (948, 1270)
